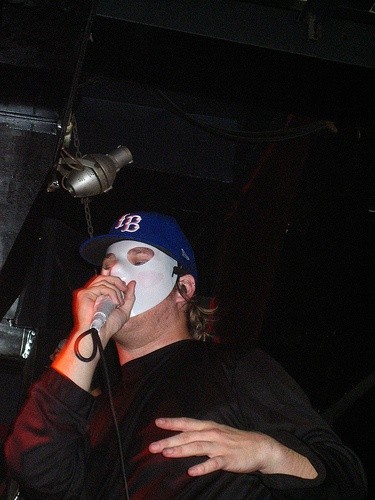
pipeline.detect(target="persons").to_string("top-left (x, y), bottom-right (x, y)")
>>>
top-left (3, 210), bottom-right (368, 500)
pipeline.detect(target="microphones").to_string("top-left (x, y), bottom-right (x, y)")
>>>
top-left (90, 291), bottom-right (125, 331)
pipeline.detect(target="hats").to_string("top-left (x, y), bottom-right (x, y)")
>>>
top-left (79, 210), bottom-right (198, 278)
